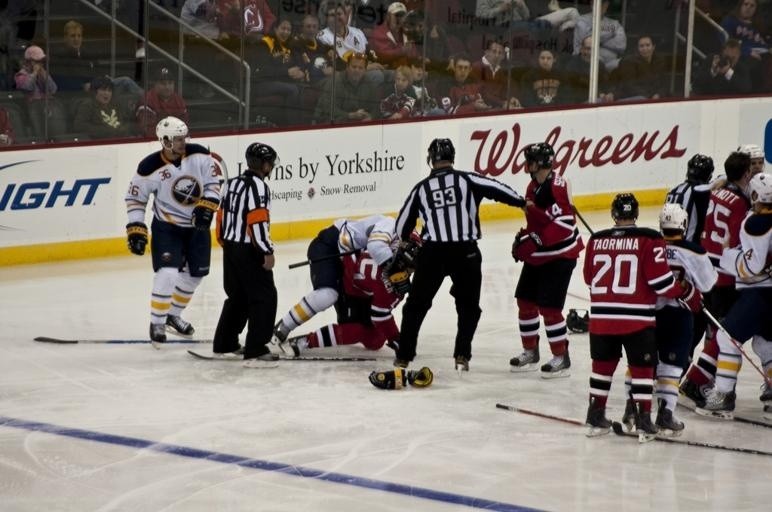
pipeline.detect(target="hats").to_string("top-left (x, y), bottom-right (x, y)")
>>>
top-left (386, 1), bottom-right (409, 16)
top-left (23, 45), bottom-right (48, 62)
top-left (153, 64), bottom-right (178, 82)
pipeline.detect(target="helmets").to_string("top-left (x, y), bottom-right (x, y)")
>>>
top-left (737, 143), bottom-right (766, 160)
top-left (426, 137), bottom-right (456, 170)
top-left (566, 307), bottom-right (591, 335)
top-left (523, 142), bottom-right (555, 176)
top-left (747, 172), bottom-right (772, 208)
top-left (685, 153), bottom-right (715, 185)
top-left (391, 230), bottom-right (422, 275)
top-left (244, 142), bottom-right (278, 181)
top-left (658, 202), bottom-right (690, 236)
top-left (611, 192), bottom-right (640, 224)
top-left (154, 115), bottom-right (190, 154)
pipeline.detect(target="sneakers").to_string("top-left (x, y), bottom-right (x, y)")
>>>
top-left (622, 398), bottom-right (639, 432)
top-left (759, 393), bottom-right (772, 423)
top-left (148, 320), bottom-right (167, 351)
top-left (165, 314), bottom-right (195, 340)
top-left (242, 353), bottom-right (281, 369)
top-left (634, 412), bottom-right (660, 445)
top-left (213, 344), bottom-right (246, 361)
top-left (392, 356), bottom-right (410, 369)
top-left (454, 355), bottom-right (470, 380)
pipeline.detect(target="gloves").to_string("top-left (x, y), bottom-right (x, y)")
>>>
top-left (368, 368), bottom-right (408, 389)
top-left (386, 261), bottom-right (412, 296)
top-left (677, 282), bottom-right (706, 315)
top-left (510, 227), bottom-right (542, 264)
top-left (124, 221), bottom-right (149, 256)
top-left (408, 366), bottom-right (433, 388)
top-left (385, 331), bottom-right (417, 362)
top-left (189, 196), bottom-right (221, 232)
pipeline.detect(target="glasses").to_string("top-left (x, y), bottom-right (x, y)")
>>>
top-left (456, 65), bottom-right (473, 73)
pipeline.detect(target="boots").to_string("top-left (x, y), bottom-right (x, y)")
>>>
top-left (509, 349), bottom-right (541, 374)
top-left (694, 388), bottom-right (737, 422)
top-left (271, 319), bottom-right (291, 349)
top-left (540, 353), bottom-right (573, 380)
top-left (654, 397), bottom-right (686, 438)
top-left (278, 335), bottom-right (310, 358)
top-left (583, 404), bottom-right (614, 438)
top-left (677, 377), bottom-right (708, 412)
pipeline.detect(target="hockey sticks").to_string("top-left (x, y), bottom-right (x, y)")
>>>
top-left (187, 350), bottom-right (379, 361)
top-left (32, 337), bottom-right (212, 344)
top-left (611, 421), bottom-right (771, 457)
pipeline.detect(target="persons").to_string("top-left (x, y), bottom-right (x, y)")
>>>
top-left (124, 116), bottom-right (230, 345)
top-left (211, 142), bottom-right (281, 364)
top-left (1, 1), bottom-right (771, 150)
top-left (268, 208), bottom-right (427, 364)
top-left (505, 139), bottom-right (586, 376)
top-left (395, 136), bottom-right (533, 371)
top-left (581, 145), bottom-right (771, 439)
